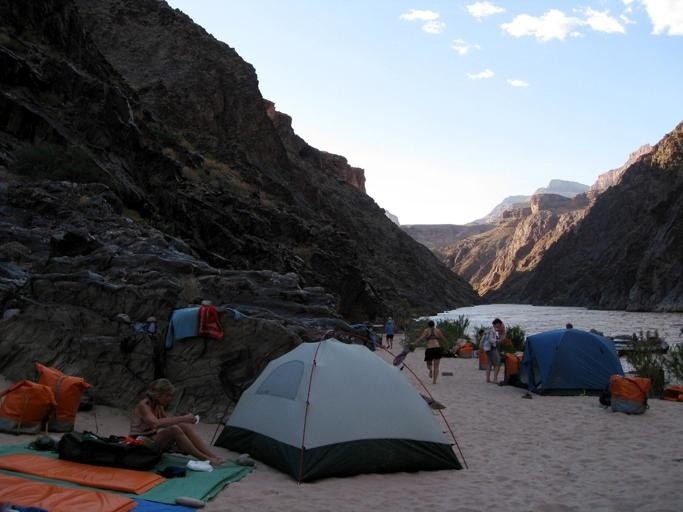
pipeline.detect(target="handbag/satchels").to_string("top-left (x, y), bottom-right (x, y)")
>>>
top-left (57, 430), bottom-right (163, 471)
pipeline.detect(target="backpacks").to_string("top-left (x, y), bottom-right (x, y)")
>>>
top-left (496, 350), bottom-right (525, 387)
top-left (458, 343), bottom-right (473, 358)
top-left (609, 373), bottom-right (651, 415)
top-left (0, 360), bottom-right (93, 434)
top-left (27, 436), bottom-right (54, 451)
top-left (478, 348), bottom-right (490, 370)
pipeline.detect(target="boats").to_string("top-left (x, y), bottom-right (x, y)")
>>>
top-left (613, 337), bottom-right (669, 352)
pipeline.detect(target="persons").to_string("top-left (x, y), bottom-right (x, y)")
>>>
top-left (565, 323), bottom-right (573, 329)
top-left (477, 328), bottom-right (485, 336)
top-left (383, 317), bottom-right (397, 350)
top-left (483, 317), bottom-right (512, 384)
top-left (409, 319), bottom-right (451, 384)
top-left (126, 378), bottom-right (227, 469)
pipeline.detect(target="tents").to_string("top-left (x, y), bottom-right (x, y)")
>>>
top-left (209, 327), bottom-right (469, 484)
top-left (513, 328), bottom-right (624, 397)
